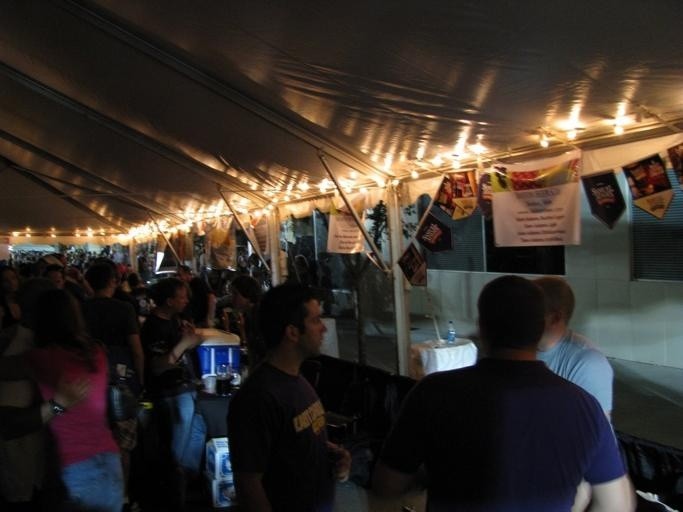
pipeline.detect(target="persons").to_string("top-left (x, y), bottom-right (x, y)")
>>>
top-left (1, 249), bottom-right (352, 512)
top-left (368, 275), bottom-right (636, 512)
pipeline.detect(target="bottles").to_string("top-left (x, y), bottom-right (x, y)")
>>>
top-left (447, 320), bottom-right (456, 345)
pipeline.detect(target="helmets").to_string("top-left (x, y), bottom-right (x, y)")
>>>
top-left (34, 254), bottom-right (65, 276)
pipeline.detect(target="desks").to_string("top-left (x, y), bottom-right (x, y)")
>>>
top-left (410, 338), bottom-right (478, 380)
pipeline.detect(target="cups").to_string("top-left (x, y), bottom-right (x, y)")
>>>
top-left (217, 377), bottom-right (234, 393)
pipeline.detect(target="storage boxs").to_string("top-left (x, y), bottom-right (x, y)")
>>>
top-left (201, 470), bottom-right (239, 508)
top-left (194, 328), bottom-right (242, 377)
top-left (207, 438), bottom-right (233, 479)
